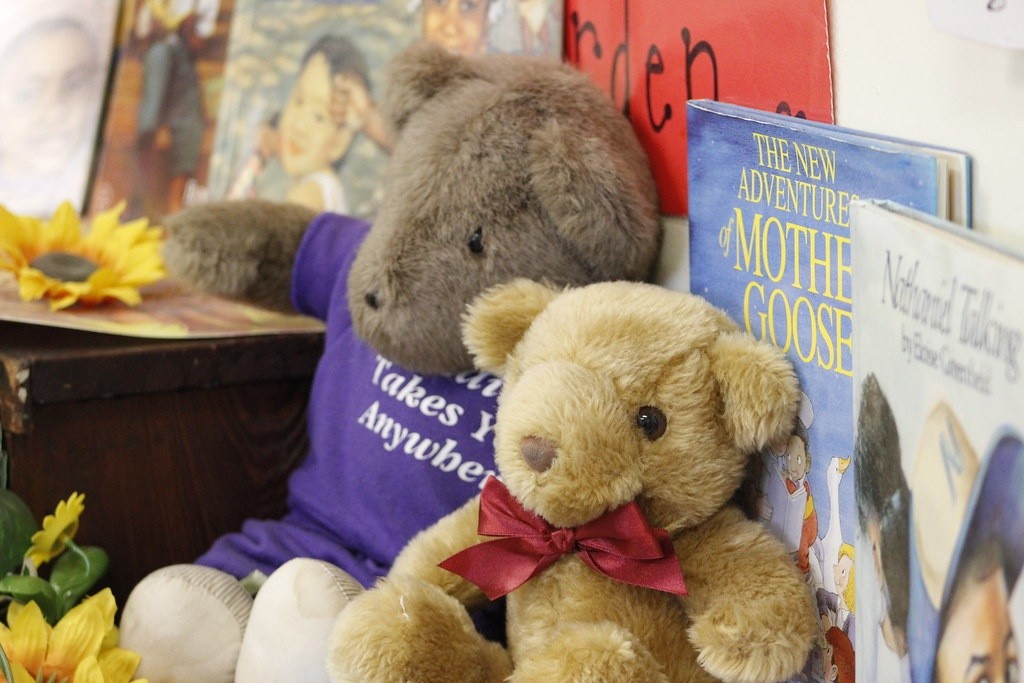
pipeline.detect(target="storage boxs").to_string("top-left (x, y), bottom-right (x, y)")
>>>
top-left (0, 269), bottom-right (332, 632)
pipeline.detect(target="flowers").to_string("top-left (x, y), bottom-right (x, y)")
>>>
top-left (0, 197), bottom-right (168, 311)
top-left (0, 492), bottom-right (148, 683)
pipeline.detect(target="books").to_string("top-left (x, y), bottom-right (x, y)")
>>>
top-left (683, 96), bottom-right (1024, 683)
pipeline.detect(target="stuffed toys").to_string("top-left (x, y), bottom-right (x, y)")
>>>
top-left (114, 41), bottom-right (822, 683)
